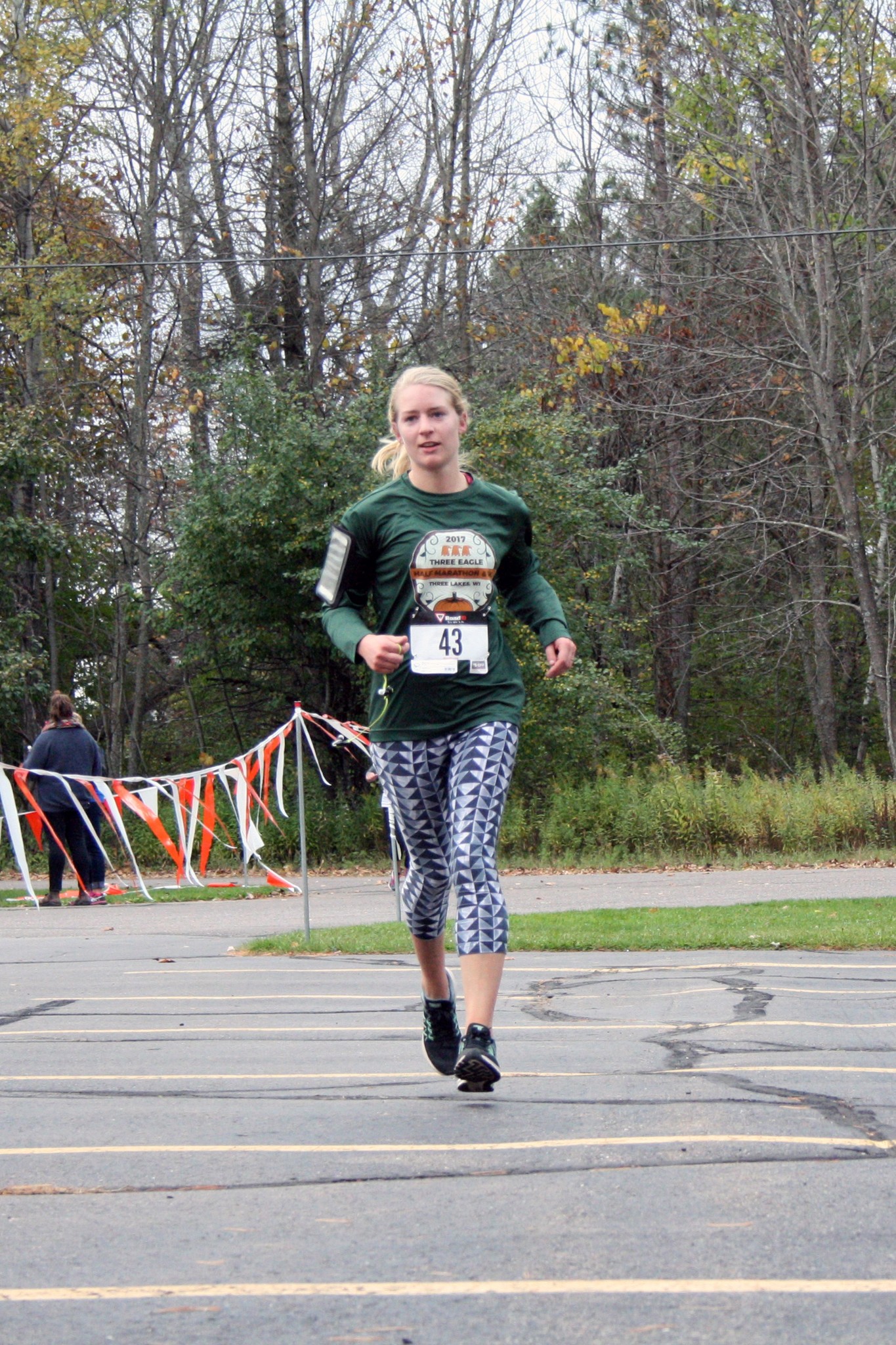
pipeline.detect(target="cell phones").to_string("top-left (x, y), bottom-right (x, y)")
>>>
top-left (317, 531), bottom-right (347, 601)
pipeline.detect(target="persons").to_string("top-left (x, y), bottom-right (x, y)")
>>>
top-left (315, 365), bottom-right (576, 1093)
top-left (17, 691), bottom-right (108, 906)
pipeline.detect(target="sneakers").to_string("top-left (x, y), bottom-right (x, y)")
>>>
top-left (36, 893), bottom-right (62, 906)
top-left (67, 893), bottom-right (91, 905)
top-left (421, 967), bottom-right (461, 1075)
top-left (86, 892), bottom-right (107, 904)
top-left (454, 1023), bottom-right (501, 1091)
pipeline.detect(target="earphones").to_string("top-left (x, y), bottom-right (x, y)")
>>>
top-left (332, 734), bottom-right (343, 746)
top-left (379, 685), bottom-right (394, 696)
